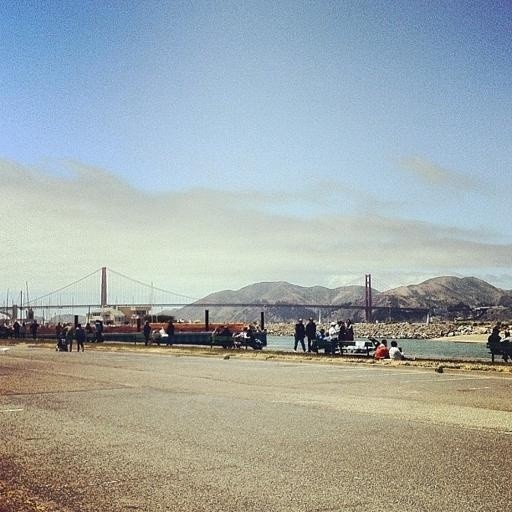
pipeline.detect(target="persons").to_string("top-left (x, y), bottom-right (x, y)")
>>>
top-left (502, 330), bottom-right (512, 342)
top-left (388, 341), bottom-right (415, 360)
top-left (487, 328), bottom-right (509, 362)
top-left (232, 329), bottom-right (243, 350)
top-left (374, 339), bottom-right (388, 355)
top-left (235, 326), bottom-right (250, 338)
top-left (292, 317), bottom-right (353, 353)
top-left (247, 326), bottom-right (257, 337)
top-left (0, 318), bottom-right (39, 341)
top-left (375, 346), bottom-right (390, 359)
top-left (143, 321), bottom-right (151, 346)
top-left (55, 319), bottom-right (104, 353)
top-left (165, 319), bottom-right (175, 346)
top-left (218, 323), bottom-right (233, 350)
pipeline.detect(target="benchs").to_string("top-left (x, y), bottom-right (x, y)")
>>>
top-left (486, 342), bottom-right (512, 362)
top-left (131, 333), bottom-right (170, 346)
top-left (311, 339), bottom-right (375, 358)
top-left (208, 336), bottom-right (250, 349)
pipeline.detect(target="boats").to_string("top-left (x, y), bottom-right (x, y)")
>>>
top-left (26, 306), bottom-right (268, 350)
top-left (334, 339), bottom-right (404, 359)
